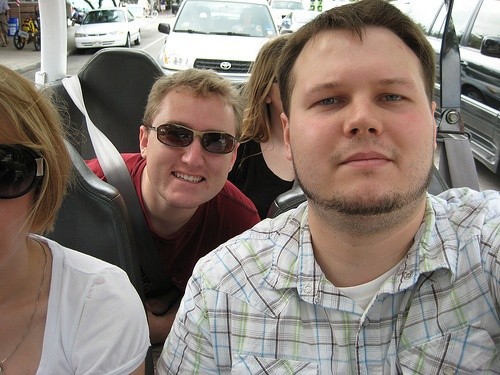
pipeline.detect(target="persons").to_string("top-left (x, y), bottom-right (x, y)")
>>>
top-left (155, 0), bottom-right (500, 375)
top-left (227, 34), bottom-right (297, 222)
top-left (0, 64), bottom-right (151, 375)
top-left (0, 0), bottom-right (11, 47)
top-left (78, 69), bottom-right (260, 366)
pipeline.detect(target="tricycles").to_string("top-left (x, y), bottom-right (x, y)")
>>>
top-left (13, 7), bottom-right (41, 51)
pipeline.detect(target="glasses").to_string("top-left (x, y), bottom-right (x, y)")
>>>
top-left (0, 143), bottom-right (43, 199)
top-left (146, 122), bottom-right (237, 155)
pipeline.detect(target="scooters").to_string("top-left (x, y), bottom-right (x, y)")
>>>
top-left (70, 2), bottom-right (89, 26)
top-left (142, 3), bottom-right (158, 19)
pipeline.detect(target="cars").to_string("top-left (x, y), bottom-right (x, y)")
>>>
top-left (385, 0), bottom-right (500, 179)
top-left (157, 0), bottom-right (295, 91)
top-left (73, 6), bottom-right (141, 51)
top-left (268, 0), bottom-right (311, 30)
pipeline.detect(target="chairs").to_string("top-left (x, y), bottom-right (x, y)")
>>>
top-left (41, 48), bottom-right (318, 299)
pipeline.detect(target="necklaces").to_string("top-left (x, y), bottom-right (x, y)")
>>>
top-left (0, 238), bottom-right (50, 372)
